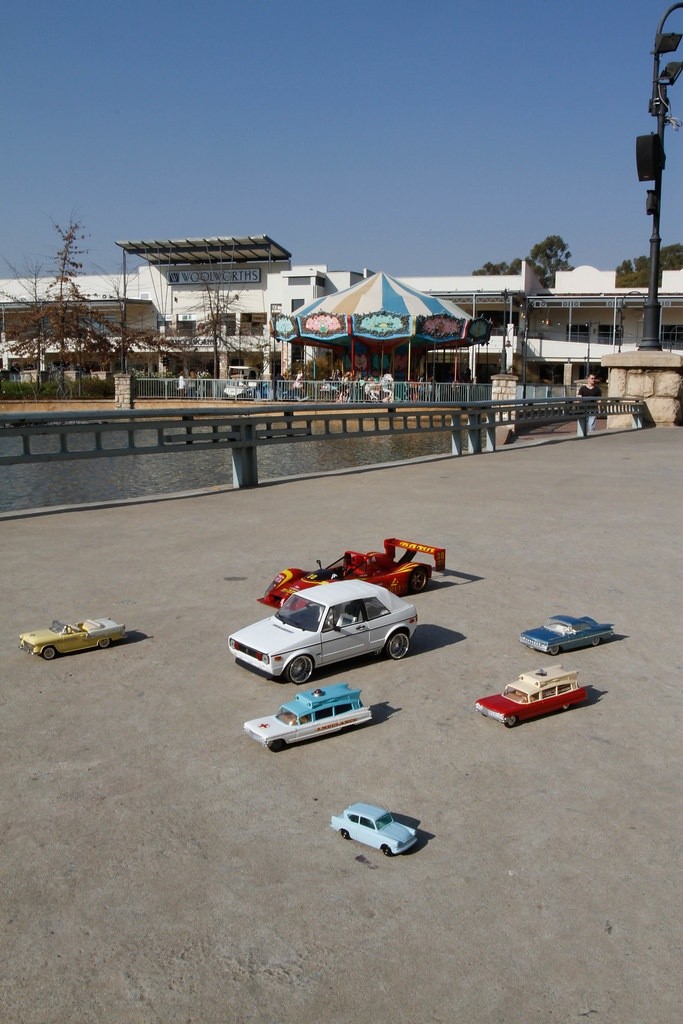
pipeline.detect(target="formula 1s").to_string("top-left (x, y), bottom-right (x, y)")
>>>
top-left (255, 537), bottom-right (447, 613)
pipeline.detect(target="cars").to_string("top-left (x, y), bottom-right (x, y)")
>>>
top-left (226, 579), bottom-right (419, 685)
top-left (519, 614), bottom-right (616, 656)
top-left (330, 801), bottom-right (419, 857)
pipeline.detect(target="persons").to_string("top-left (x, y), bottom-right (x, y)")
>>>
top-left (177, 368), bottom-right (472, 403)
top-left (577, 374), bottom-right (602, 435)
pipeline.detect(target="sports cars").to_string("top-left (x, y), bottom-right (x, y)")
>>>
top-left (17, 615), bottom-right (130, 661)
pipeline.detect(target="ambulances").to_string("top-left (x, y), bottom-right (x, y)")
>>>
top-left (242, 680), bottom-right (374, 753)
top-left (472, 664), bottom-right (589, 729)
